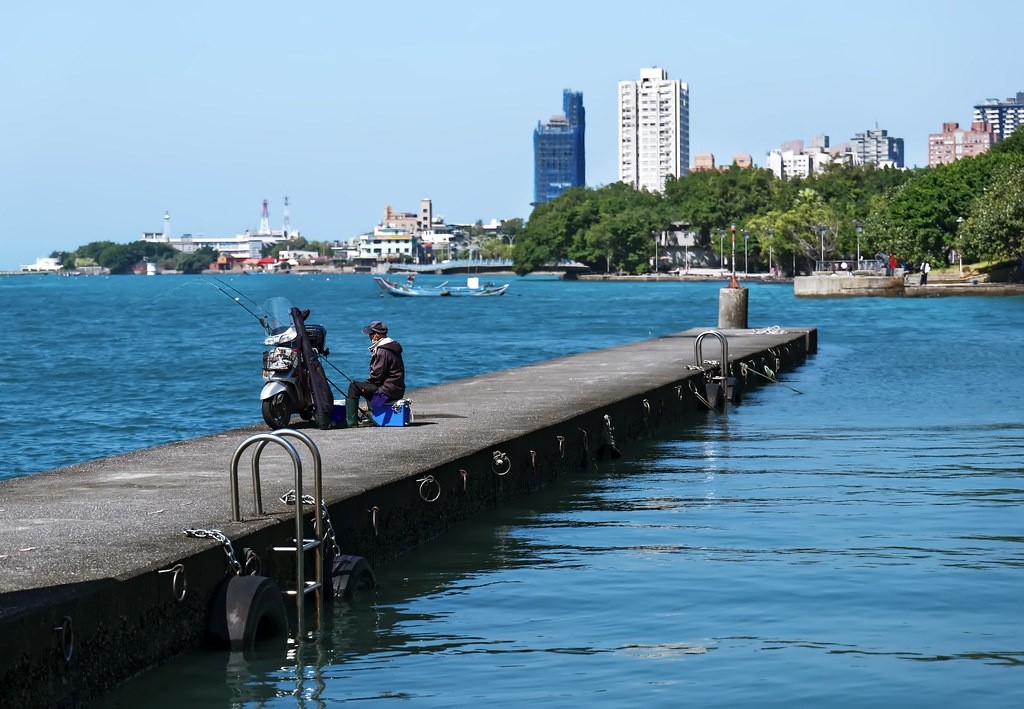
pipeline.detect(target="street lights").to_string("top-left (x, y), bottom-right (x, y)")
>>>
top-left (956, 215), bottom-right (969, 279)
top-left (716, 227), bottom-right (725, 270)
top-left (740, 229), bottom-right (750, 273)
top-left (821, 228), bottom-right (827, 271)
top-left (766, 227), bottom-right (774, 273)
top-left (682, 228), bottom-right (690, 271)
top-left (855, 227), bottom-right (863, 270)
top-left (650, 230), bottom-right (660, 274)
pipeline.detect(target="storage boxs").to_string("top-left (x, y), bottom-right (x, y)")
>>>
top-left (368, 398), bottom-right (412, 426)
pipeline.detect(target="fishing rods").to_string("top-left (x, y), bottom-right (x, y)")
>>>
top-left (198, 272), bottom-right (380, 428)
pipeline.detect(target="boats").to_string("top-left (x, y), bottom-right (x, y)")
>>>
top-left (370, 274), bottom-right (510, 298)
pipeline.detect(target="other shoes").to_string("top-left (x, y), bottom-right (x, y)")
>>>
top-left (336, 421), bottom-right (358, 428)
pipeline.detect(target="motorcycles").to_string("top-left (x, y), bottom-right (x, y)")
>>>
top-left (257, 297), bottom-right (327, 431)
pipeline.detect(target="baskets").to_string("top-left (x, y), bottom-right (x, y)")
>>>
top-left (263, 350), bottom-right (296, 372)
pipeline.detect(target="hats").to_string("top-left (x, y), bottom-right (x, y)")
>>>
top-left (362, 321), bottom-right (388, 334)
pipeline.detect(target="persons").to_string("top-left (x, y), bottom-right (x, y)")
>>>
top-left (884, 253), bottom-right (896, 277)
top-left (919, 260), bottom-right (931, 284)
top-left (407, 274), bottom-right (415, 286)
top-left (834, 262), bottom-right (853, 272)
top-left (345, 321), bottom-right (406, 428)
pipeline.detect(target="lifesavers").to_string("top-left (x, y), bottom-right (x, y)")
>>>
top-left (696, 376), bottom-right (745, 410)
top-left (323, 554), bottom-right (379, 601)
top-left (200, 575), bottom-right (289, 653)
